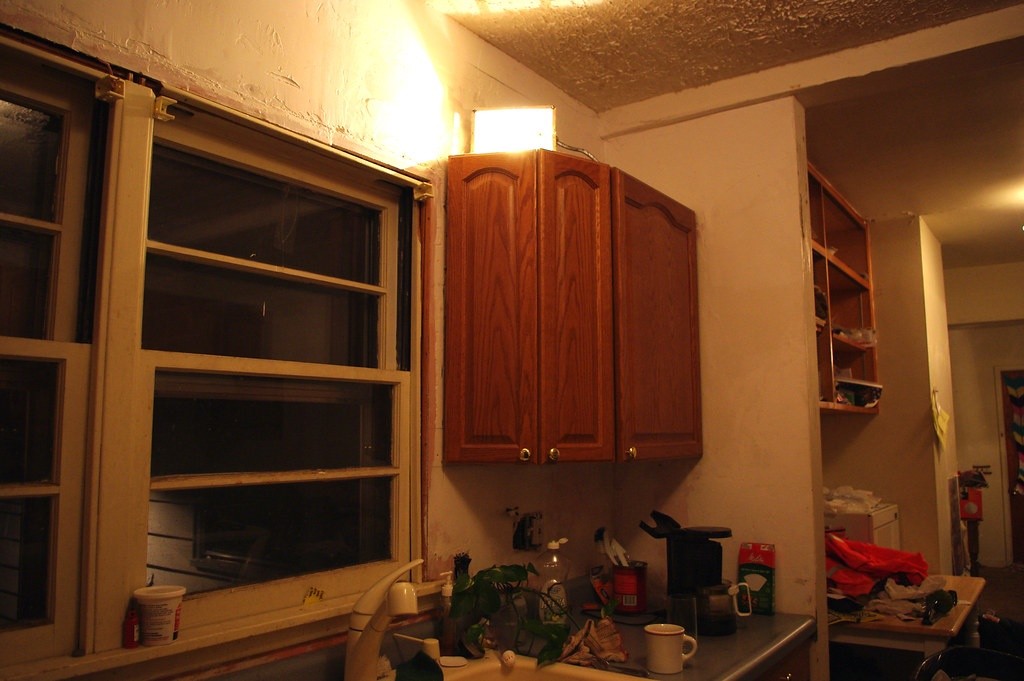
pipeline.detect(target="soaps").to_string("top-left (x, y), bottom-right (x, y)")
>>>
top-left (438, 656), bottom-right (468, 667)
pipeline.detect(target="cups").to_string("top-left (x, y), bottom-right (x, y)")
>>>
top-left (133, 585), bottom-right (186, 646)
top-left (645, 622), bottom-right (698, 675)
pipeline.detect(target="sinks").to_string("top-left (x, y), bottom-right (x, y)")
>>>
top-left (376, 645), bottom-right (662, 681)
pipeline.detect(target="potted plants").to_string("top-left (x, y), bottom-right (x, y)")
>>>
top-left (448, 563), bottom-right (620, 668)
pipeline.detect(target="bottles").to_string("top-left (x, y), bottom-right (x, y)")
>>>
top-left (535, 537), bottom-right (569, 630)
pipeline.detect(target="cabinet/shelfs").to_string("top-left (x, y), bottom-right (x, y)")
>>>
top-left (807, 160), bottom-right (883, 414)
top-left (755, 641), bottom-right (815, 681)
top-left (443, 149), bottom-right (703, 465)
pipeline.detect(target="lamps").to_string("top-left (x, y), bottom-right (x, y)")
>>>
top-left (470, 105), bottom-right (599, 163)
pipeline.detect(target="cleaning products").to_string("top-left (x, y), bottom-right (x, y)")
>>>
top-left (539, 538), bottom-right (569, 623)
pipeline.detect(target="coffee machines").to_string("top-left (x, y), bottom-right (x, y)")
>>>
top-left (640, 510), bottom-right (753, 638)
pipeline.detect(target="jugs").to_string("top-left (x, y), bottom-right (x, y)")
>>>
top-left (684, 577), bottom-right (752, 641)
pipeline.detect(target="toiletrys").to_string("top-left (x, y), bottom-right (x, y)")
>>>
top-left (439, 572), bottom-right (456, 655)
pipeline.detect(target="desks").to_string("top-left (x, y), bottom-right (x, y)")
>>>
top-left (827, 571), bottom-right (987, 660)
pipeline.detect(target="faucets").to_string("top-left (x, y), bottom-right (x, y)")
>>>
top-left (343, 559), bottom-right (425, 681)
